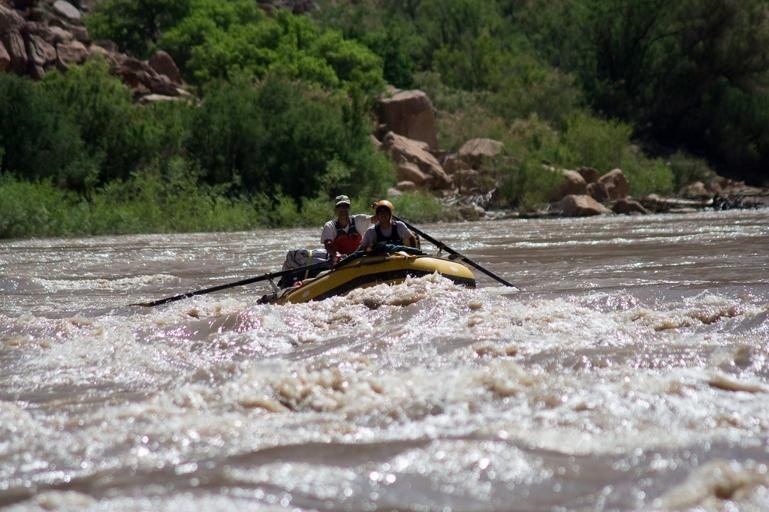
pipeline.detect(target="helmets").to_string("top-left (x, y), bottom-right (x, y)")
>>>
top-left (374, 199), bottom-right (395, 215)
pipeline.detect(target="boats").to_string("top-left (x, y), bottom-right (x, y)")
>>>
top-left (257, 250), bottom-right (476, 305)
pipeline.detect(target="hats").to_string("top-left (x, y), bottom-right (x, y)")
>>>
top-left (334, 194), bottom-right (352, 208)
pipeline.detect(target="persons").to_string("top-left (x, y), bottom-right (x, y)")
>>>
top-left (320, 192), bottom-right (379, 268)
top-left (355, 198), bottom-right (418, 259)
